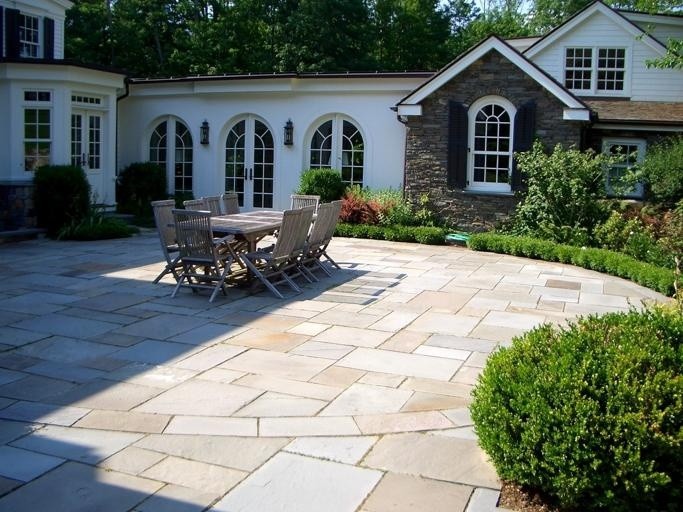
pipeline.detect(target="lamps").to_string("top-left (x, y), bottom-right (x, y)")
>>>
top-left (281, 118), bottom-right (293, 145)
top-left (198, 118), bottom-right (209, 146)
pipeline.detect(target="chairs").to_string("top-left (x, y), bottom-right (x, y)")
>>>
top-left (149, 192), bottom-right (345, 306)
